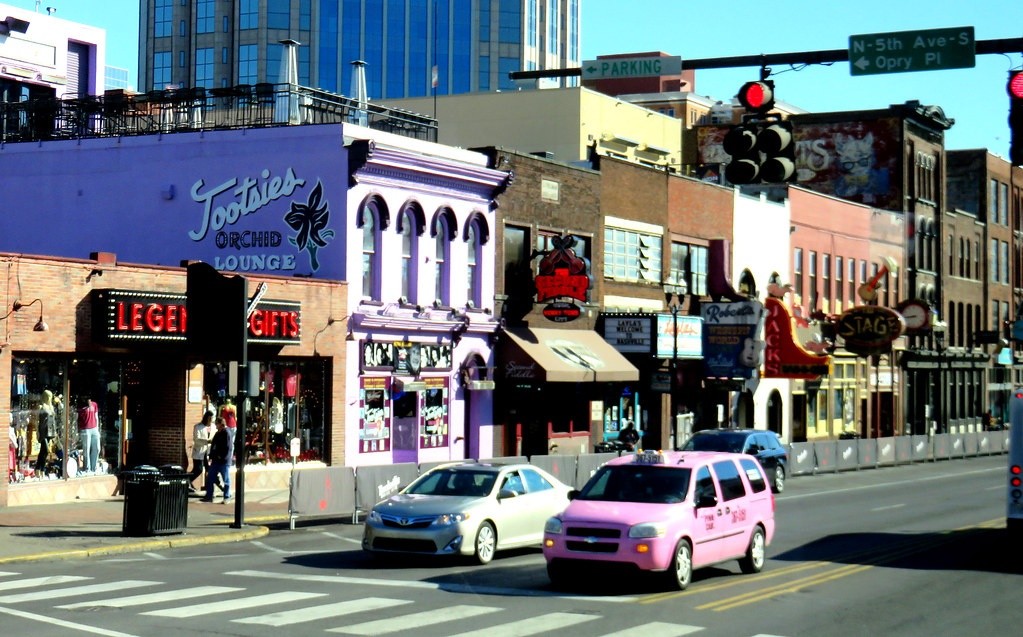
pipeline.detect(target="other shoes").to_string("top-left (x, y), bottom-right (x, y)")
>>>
top-left (217, 484), bottom-right (224, 491)
top-left (200, 497), bottom-right (213, 502)
top-left (222, 498), bottom-right (234, 506)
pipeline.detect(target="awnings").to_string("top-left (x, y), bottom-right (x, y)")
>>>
top-left (504, 327), bottom-right (640, 382)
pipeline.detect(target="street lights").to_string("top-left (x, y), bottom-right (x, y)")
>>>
top-left (658, 277), bottom-right (689, 452)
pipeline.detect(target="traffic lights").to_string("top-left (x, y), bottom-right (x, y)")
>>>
top-left (1004, 70), bottom-right (1023, 168)
top-left (722, 124), bottom-right (760, 184)
top-left (736, 79), bottom-right (775, 112)
top-left (758, 122), bottom-right (796, 184)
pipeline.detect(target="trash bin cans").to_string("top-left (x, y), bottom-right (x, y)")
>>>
top-left (120, 463), bottom-right (193, 535)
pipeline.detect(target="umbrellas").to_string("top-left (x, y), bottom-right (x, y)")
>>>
top-left (346, 59), bottom-right (370, 109)
top-left (275, 40), bottom-right (312, 123)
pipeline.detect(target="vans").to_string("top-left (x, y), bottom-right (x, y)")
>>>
top-left (543, 449), bottom-right (776, 594)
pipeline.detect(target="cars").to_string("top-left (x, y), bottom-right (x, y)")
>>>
top-left (677, 427), bottom-right (790, 494)
top-left (360, 461), bottom-right (576, 566)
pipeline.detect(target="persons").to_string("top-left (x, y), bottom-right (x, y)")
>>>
top-left (8, 412), bottom-right (18, 482)
top-left (32, 389), bottom-right (57, 478)
top-left (364, 345), bottom-right (448, 371)
top-left (218, 398), bottom-right (238, 466)
top-left (78, 399), bottom-right (101, 471)
top-left (271, 398), bottom-right (283, 448)
top-left (199, 418), bottom-right (233, 504)
top-left (187, 411), bottom-right (219, 492)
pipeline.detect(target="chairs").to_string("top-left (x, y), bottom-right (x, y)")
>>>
top-left (0, 82), bottom-right (276, 143)
top-left (298, 86), bottom-right (431, 140)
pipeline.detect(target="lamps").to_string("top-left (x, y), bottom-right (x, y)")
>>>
top-left (458, 315), bottom-right (471, 335)
top-left (329, 314), bottom-right (357, 342)
top-left (14, 299), bottom-right (50, 332)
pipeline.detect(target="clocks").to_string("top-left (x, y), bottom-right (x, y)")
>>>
top-left (902, 305), bottom-right (925, 328)
